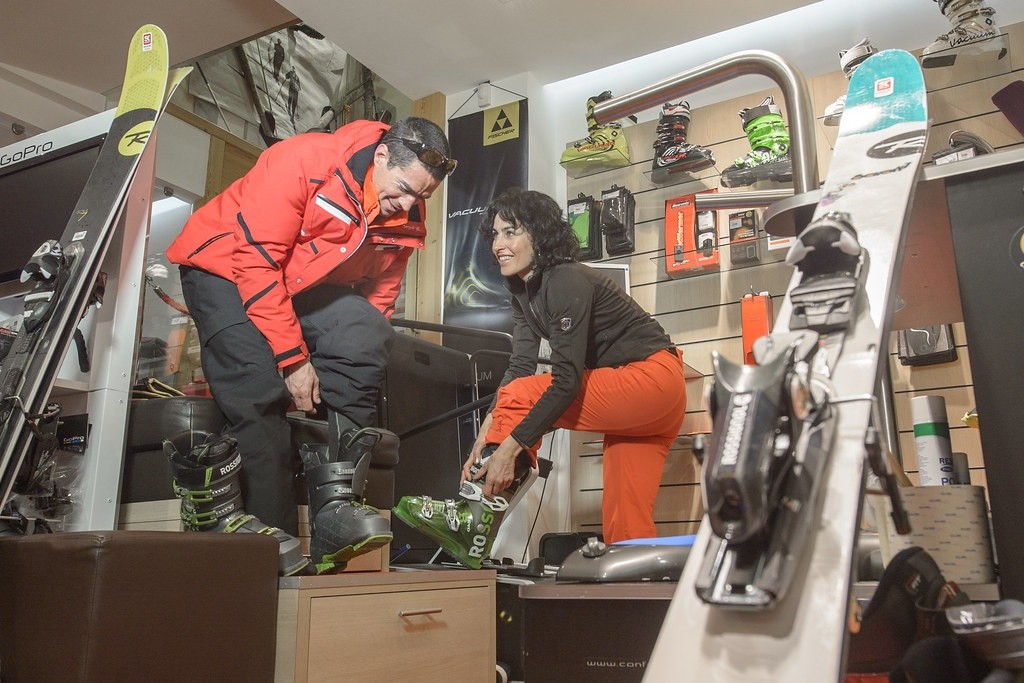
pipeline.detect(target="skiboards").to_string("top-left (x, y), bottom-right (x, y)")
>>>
top-left (0, 23), bottom-right (194, 512)
top-left (635, 49), bottom-right (931, 683)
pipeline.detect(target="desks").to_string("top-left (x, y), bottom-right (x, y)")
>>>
top-left (30, 377), bottom-right (89, 478)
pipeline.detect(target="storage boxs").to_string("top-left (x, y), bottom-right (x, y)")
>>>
top-left (767, 232), bottom-right (797, 250)
top-left (663, 187), bottom-right (721, 279)
top-left (274, 569), bottom-right (496, 683)
top-left (727, 210), bottom-right (761, 265)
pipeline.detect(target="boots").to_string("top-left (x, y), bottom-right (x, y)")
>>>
top-left (162, 434), bottom-right (307, 577)
top-left (298, 425), bottom-right (393, 572)
top-left (393, 447), bottom-right (531, 570)
top-left (652, 105), bottom-right (714, 184)
top-left (719, 101), bottom-right (794, 187)
top-left (559, 91), bottom-right (629, 166)
top-left (920, 0), bottom-right (1007, 70)
top-left (822, 40), bottom-right (880, 127)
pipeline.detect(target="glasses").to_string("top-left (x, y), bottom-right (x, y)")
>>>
top-left (390, 137), bottom-right (458, 177)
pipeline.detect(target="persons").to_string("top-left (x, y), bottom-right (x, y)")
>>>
top-left (166, 116), bottom-right (459, 577)
top-left (393, 187), bottom-right (687, 570)
